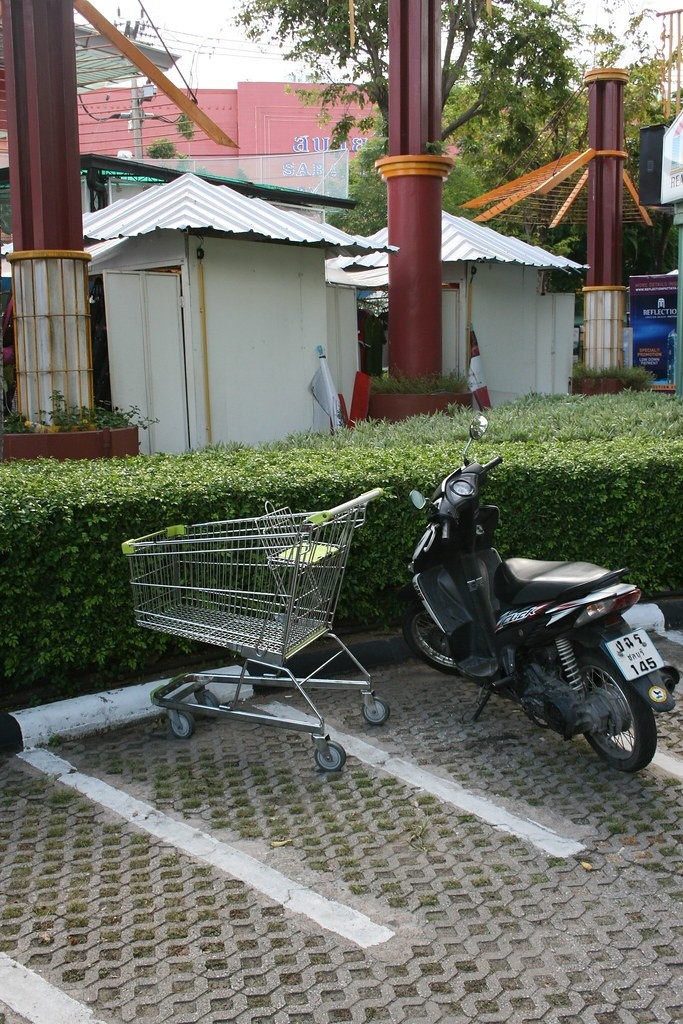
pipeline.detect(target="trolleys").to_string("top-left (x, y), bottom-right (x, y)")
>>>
top-left (121, 485), bottom-right (389, 774)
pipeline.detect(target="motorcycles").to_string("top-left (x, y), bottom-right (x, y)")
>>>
top-left (397, 414), bottom-right (681, 772)
